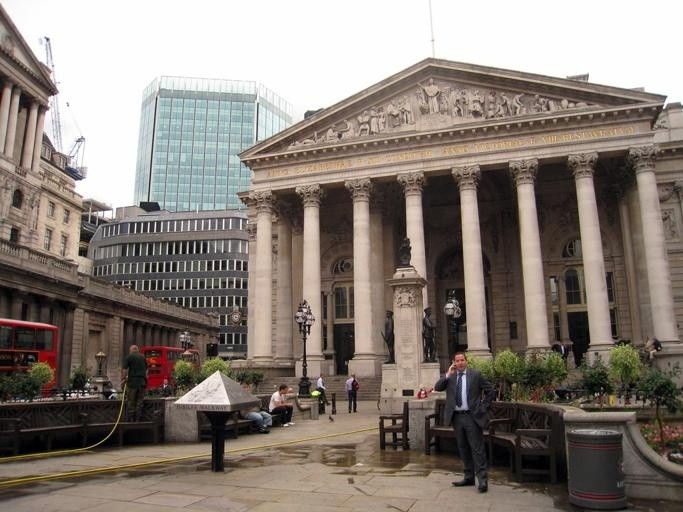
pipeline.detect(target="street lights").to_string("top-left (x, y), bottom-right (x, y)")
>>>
top-left (443, 288), bottom-right (462, 359)
top-left (93, 350), bottom-right (108, 377)
top-left (180, 330), bottom-right (193, 350)
top-left (296, 300), bottom-right (316, 397)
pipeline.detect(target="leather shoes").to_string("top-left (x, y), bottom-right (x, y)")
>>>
top-left (479, 480), bottom-right (487, 490)
top-left (452, 478), bottom-right (475, 486)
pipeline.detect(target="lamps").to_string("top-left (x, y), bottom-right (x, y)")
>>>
top-left (443, 289), bottom-right (455, 315)
top-left (450, 290), bottom-right (461, 319)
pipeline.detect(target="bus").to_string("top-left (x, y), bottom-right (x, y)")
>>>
top-left (138, 345), bottom-right (201, 394)
top-left (0, 317), bottom-right (59, 398)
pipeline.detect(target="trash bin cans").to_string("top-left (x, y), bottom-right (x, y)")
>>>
top-left (564, 428), bottom-right (627, 512)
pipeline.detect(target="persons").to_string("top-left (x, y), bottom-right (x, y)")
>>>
top-left (236, 383), bottom-right (272, 433)
top-left (557, 341), bottom-right (569, 368)
top-left (381, 310), bottom-right (397, 364)
top-left (637, 337), bottom-right (663, 366)
top-left (270, 385), bottom-right (296, 428)
top-left (344, 373), bottom-right (358, 414)
top-left (286, 76), bottom-right (591, 150)
top-left (157, 379), bottom-right (171, 396)
top-left (421, 307), bottom-right (436, 362)
top-left (122, 345), bottom-right (148, 423)
top-left (316, 373), bottom-right (329, 407)
top-left (433, 353), bottom-right (494, 493)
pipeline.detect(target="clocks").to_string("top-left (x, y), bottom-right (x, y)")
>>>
top-left (230, 311), bottom-right (247, 329)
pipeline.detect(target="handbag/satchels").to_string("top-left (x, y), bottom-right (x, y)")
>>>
top-left (352, 379), bottom-right (359, 390)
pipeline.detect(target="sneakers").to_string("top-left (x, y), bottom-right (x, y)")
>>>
top-left (281, 422), bottom-right (294, 427)
top-left (259, 425), bottom-right (269, 433)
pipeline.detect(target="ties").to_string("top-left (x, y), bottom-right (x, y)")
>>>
top-left (456, 373), bottom-right (463, 407)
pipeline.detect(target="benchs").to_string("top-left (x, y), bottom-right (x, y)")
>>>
top-left (379, 400), bottom-right (409, 451)
top-left (489, 404), bottom-right (559, 484)
top-left (236, 395), bottom-right (282, 438)
top-left (0, 401), bottom-right (87, 457)
top-left (425, 399), bottom-right (514, 465)
top-left (82, 399), bottom-right (165, 452)
top-left (197, 411), bottom-right (237, 442)
